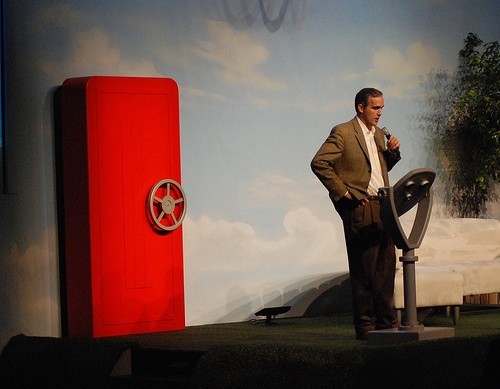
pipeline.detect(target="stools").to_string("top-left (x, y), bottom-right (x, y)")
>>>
top-left (394, 272), bottom-right (463, 323)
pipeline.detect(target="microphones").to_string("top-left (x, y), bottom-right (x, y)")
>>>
top-left (382, 127), bottom-right (400, 155)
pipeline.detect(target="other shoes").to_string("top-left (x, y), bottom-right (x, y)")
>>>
top-left (356, 332), bottom-right (368, 339)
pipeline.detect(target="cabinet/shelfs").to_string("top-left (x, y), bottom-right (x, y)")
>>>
top-left (61, 75), bottom-right (187, 339)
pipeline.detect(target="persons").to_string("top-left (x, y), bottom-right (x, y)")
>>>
top-left (310, 87), bottom-right (401, 342)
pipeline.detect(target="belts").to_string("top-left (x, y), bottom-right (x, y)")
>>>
top-left (368, 196), bottom-right (379, 200)
